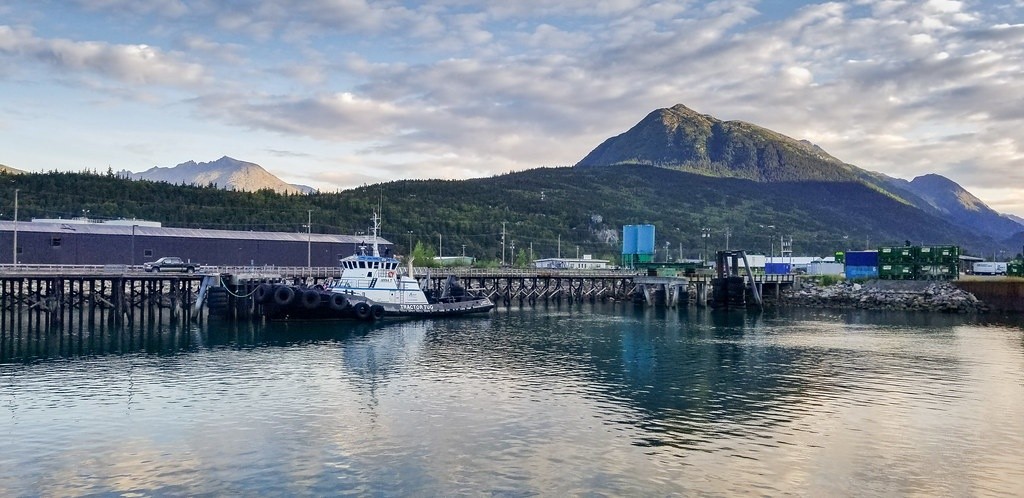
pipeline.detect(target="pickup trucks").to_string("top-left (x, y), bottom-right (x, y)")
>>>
top-left (142, 256), bottom-right (201, 274)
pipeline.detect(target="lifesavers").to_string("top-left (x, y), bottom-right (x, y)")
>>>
top-left (273, 286), bottom-right (294, 305)
top-left (352, 302), bottom-right (370, 319)
top-left (369, 303), bottom-right (386, 320)
top-left (302, 289), bottom-right (322, 307)
top-left (388, 271), bottom-right (394, 278)
top-left (329, 293), bottom-right (348, 311)
top-left (256, 285), bottom-right (273, 301)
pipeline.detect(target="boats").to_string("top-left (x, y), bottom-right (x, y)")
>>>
top-left (256, 211), bottom-right (496, 321)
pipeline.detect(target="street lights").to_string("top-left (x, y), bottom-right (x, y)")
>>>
top-left (12, 187), bottom-right (22, 273)
top-left (131, 224), bottom-right (139, 273)
